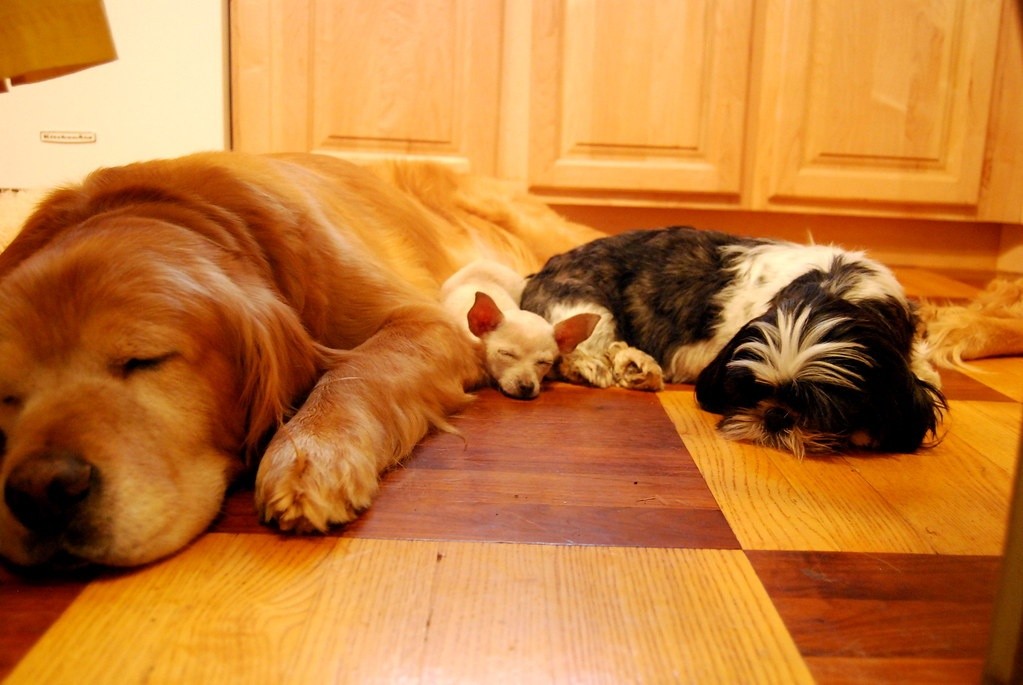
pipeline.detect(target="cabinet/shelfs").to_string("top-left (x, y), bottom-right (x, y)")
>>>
top-left (225, 1), bottom-right (1023, 280)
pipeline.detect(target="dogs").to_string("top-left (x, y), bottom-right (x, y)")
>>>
top-left (439, 260), bottom-right (600, 399)
top-left (0, 144), bottom-right (609, 570)
top-left (521, 223), bottom-right (951, 457)
